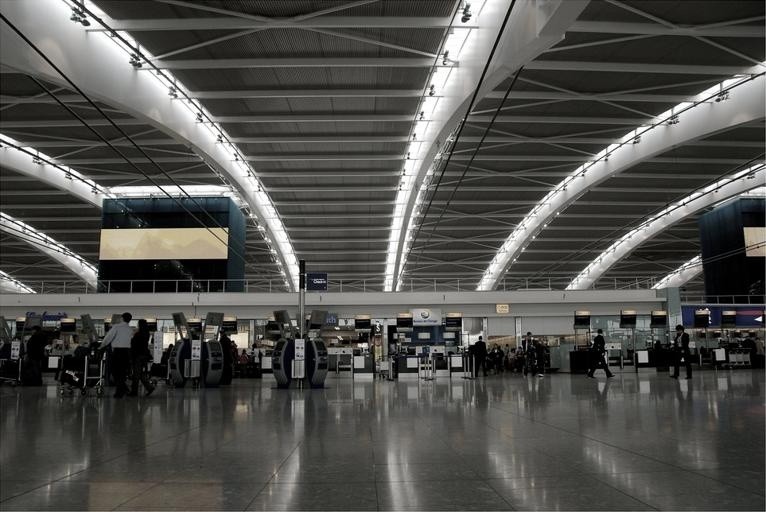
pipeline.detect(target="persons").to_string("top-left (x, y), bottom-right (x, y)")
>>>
top-left (220, 330), bottom-right (256, 385)
top-left (755, 337), bottom-right (765, 368)
top-left (588, 329), bottom-right (615, 378)
top-left (26, 313), bottom-right (174, 398)
top-left (670, 324), bottom-right (692, 379)
top-left (743, 336), bottom-right (757, 368)
top-left (472, 332), bottom-right (547, 377)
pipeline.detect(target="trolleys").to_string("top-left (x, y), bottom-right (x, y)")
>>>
top-left (57, 347), bottom-right (105, 400)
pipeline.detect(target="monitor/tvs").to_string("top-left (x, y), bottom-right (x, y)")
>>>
top-left (694, 314), bottom-right (710, 327)
top-left (651, 315), bottom-right (667, 325)
top-left (147, 322), bottom-right (157, 331)
top-left (573, 315), bottom-right (591, 326)
top-left (620, 314), bottom-right (637, 325)
top-left (396, 318), bottom-right (413, 328)
top-left (220, 321), bottom-right (237, 334)
top-left (722, 314), bottom-right (737, 325)
top-left (445, 317), bottom-right (462, 327)
top-left (60, 322), bottom-right (77, 332)
top-left (354, 319), bottom-right (371, 329)
top-left (16, 321), bottom-right (25, 331)
top-left (187, 322), bottom-right (202, 332)
